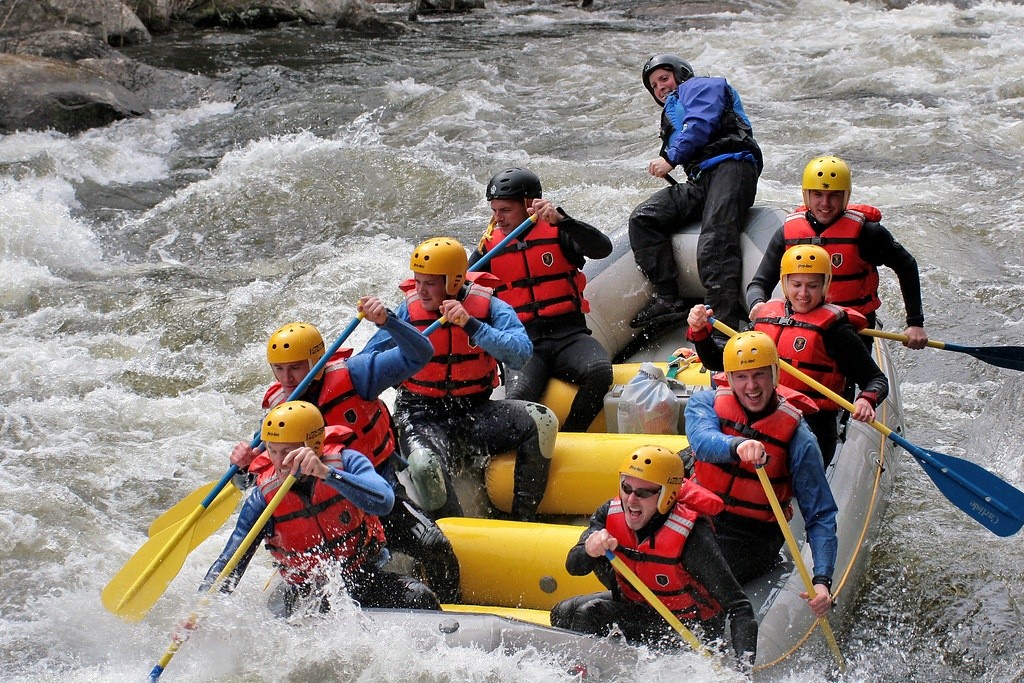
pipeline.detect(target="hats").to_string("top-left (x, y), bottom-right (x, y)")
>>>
top-left (260, 400), bottom-right (325, 459)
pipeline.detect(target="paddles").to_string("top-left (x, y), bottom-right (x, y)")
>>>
top-left (144, 313), bottom-right (454, 548)
top-left (462, 208), bottom-right (540, 275)
top-left (98, 303), bottom-right (367, 626)
top-left (858, 328), bottom-right (1024, 374)
top-left (146, 459), bottom-right (301, 682)
top-left (602, 550), bottom-right (722, 669)
top-left (701, 301), bottom-right (1024, 538)
top-left (747, 448), bottom-right (852, 683)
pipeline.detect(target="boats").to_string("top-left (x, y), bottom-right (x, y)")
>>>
top-left (255, 206), bottom-right (903, 682)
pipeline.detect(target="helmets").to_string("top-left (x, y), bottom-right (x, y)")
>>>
top-left (266, 322), bottom-right (326, 380)
top-left (642, 53), bottom-right (694, 107)
top-left (723, 331), bottom-right (780, 393)
top-left (802, 155), bottom-right (852, 212)
top-left (780, 244), bottom-right (832, 300)
top-left (485, 167), bottom-right (542, 203)
top-left (410, 236), bottom-right (467, 295)
top-left (618, 444), bottom-right (684, 516)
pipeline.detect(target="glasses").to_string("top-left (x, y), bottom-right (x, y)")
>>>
top-left (621, 480), bottom-right (661, 499)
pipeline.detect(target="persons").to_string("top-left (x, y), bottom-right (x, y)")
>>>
top-left (743, 156), bottom-right (928, 443)
top-left (550, 443), bottom-right (759, 676)
top-left (227, 296), bottom-right (462, 604)
top-left (167, 400), bottom-right (462, 644)
top-left (351, 237), bottom-right (561, 522)
top-left (464, 167), bottom-right (615, 433)
top-left (683, 331), bottom-right (840, 617)
top-left (685, 244), bottom-right (889, 473)
top-left (629, 53), bottom-right (764, 342)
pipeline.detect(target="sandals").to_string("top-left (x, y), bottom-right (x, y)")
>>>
top-left (711, 314), bottom-right (739, 339)
top-left (629, 292), bottom-right (688, 329)
